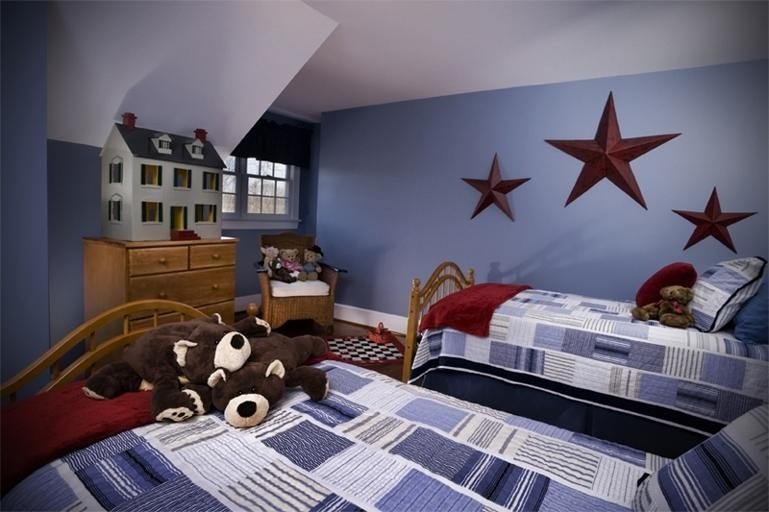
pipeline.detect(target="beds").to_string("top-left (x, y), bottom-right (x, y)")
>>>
top-left (0, 297), bottom-right (769, 512)
top-left (402, 257), bottom-right (768, 436)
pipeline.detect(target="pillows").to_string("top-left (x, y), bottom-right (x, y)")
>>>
top-left (687, 256), bottom-right (768, 333)
top-left (636, 262), bottom-right (697, 307)
top-left (731, 275), bottom-right (768, 343)
top-left (629, 406), bottom-right (768, 512)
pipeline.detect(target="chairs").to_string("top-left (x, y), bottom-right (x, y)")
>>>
top-left (256, 232), bottom-right (341, 333)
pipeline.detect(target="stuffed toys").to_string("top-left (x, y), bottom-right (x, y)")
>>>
top-left (260, 243), bottom-right (324, 282)
top-left (82, 311), bottom-right (273, 426)
top-left (183, 328), bottom-right (332, 428)
top-left (633, 283), bottom-right (697, 329)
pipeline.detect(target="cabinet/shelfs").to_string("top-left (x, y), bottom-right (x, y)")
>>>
top-left (81, 236), bottom-right (240, 378)
